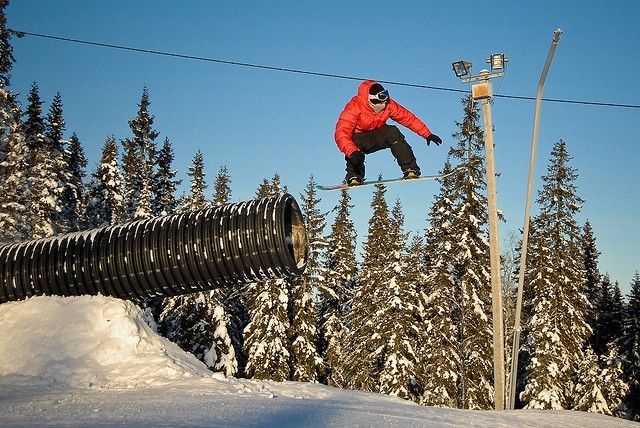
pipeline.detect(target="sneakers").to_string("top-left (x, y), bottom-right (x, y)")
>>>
top-left (404, 169), bottom-right (421, 178)
top-left (347, 176), bottom-right (364, 186)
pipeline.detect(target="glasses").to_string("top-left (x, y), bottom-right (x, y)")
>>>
top-left (369, 90), bottom-right (389, 101)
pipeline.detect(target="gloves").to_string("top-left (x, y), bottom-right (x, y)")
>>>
top-left (426, 134), bottom-right (442, 146)
top-left (353, 151), bottom-right (365, 162)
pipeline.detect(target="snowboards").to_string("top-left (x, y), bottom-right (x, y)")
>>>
top-left (316, 167), bottom-right (466, 191)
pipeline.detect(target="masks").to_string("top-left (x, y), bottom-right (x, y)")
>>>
top-left (369, 102), bottom-right (386, 112)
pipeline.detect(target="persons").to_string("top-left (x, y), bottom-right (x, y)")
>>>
top-left (334, 80), bottom-right (443, 187)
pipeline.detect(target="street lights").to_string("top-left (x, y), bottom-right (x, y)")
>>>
top-left (452, 53), bottom-right (507, 411)
